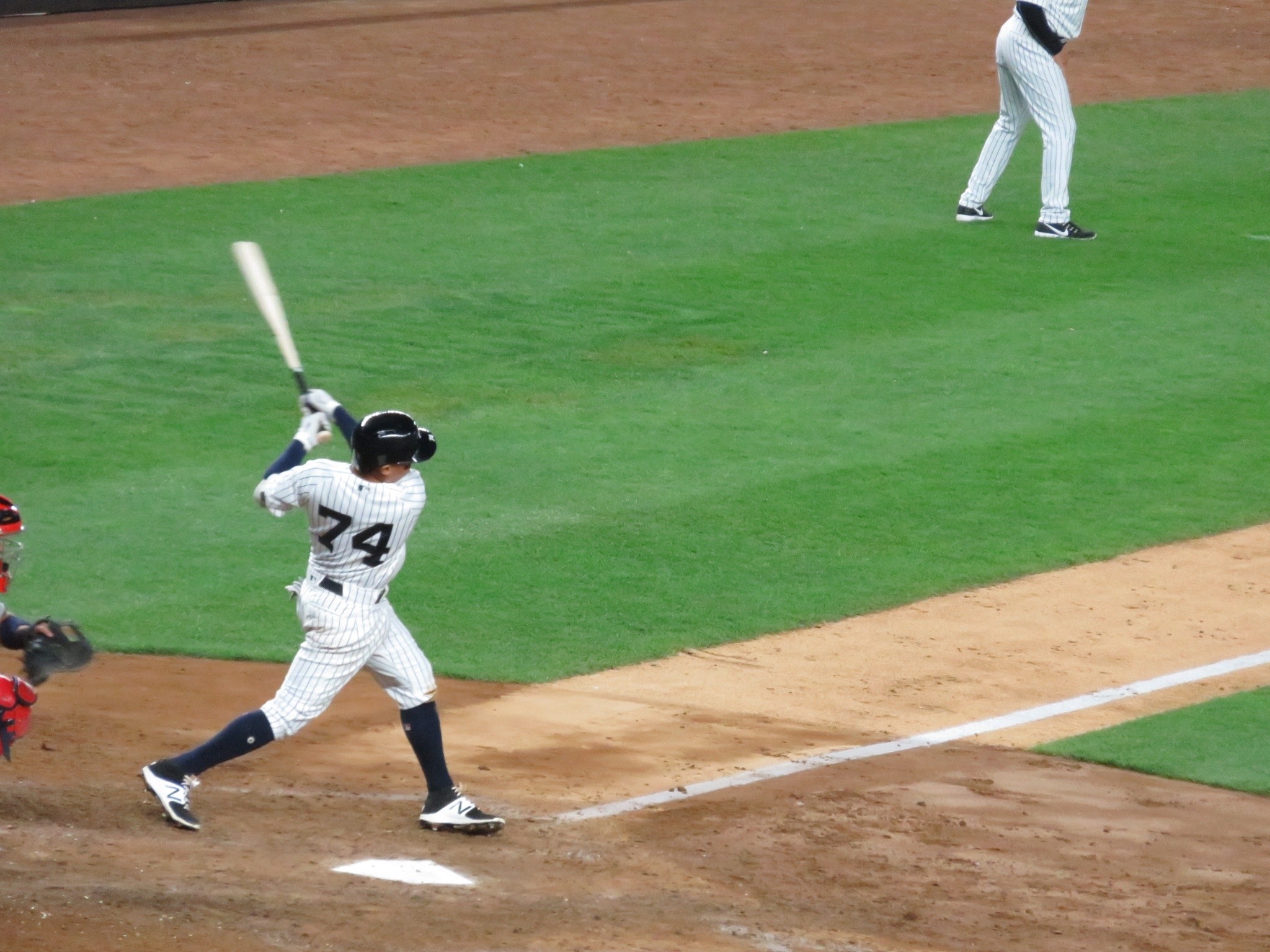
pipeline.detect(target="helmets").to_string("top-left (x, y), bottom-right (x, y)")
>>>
top-left (350, 410), bottom-right (436, 473)
top-left (0, 495), bottom-right (25, 592)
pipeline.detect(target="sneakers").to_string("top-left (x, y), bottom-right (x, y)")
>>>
top-left (1034, 219), bottom-right (1097, 240)
top-left (136, 755), bottom-right (201, 830)
top-left (416, 783), bottom-right (506, 837)
top-left (955, 202), bottom-right (994, 221)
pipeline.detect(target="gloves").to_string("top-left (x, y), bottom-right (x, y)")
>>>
top-left (293, 410), bottom-right (332, 453)
top-left (298, 388), bottom-right (341, 420)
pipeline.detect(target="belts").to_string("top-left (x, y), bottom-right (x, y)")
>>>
top-left (311, 574), bottom-right (385, 604)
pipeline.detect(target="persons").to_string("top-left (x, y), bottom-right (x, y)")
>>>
top-left (141, 389), bottom-right (506, 835)
top-left (1, 496), bottom-right (53, 758)
top-left (956, 1), bottom-right (1098, 241)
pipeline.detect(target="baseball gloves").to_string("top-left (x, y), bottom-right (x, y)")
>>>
top-left (21, 618), bottom-right (94, 687)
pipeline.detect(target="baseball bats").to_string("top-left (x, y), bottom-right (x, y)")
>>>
top-left (231, 240), bottom-right (332, 446)
